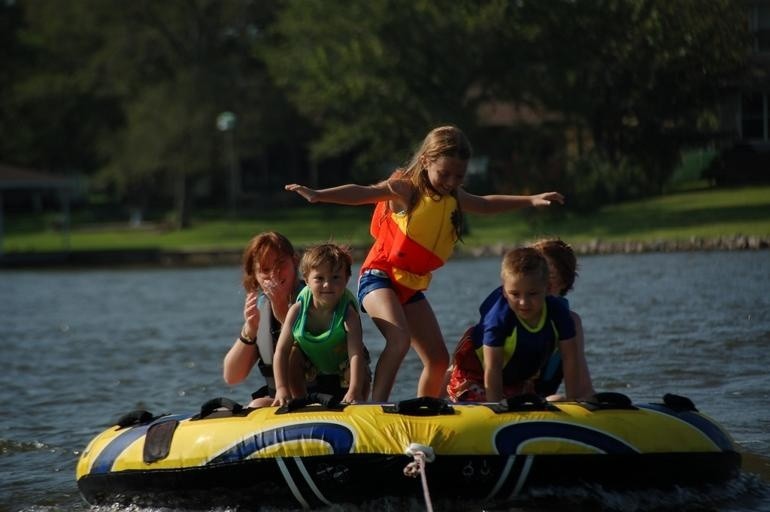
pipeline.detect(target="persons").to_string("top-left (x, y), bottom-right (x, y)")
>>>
top-left (267, 235), bottom-right (372, 409)
top-left (221, 229), bottom-right (349, 411)
top-left (282, 124), bottom-right (569, 405)
top-left (446, 244), bottom-right (584, 408)
top-left (522, 235), bottom-right (599, 405)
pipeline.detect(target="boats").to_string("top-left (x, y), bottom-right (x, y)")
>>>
top-left (76, 387), bottom-right (745, 509)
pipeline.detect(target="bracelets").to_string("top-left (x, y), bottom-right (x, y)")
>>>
top-left (237, 327), bottom-right (258, 346)
